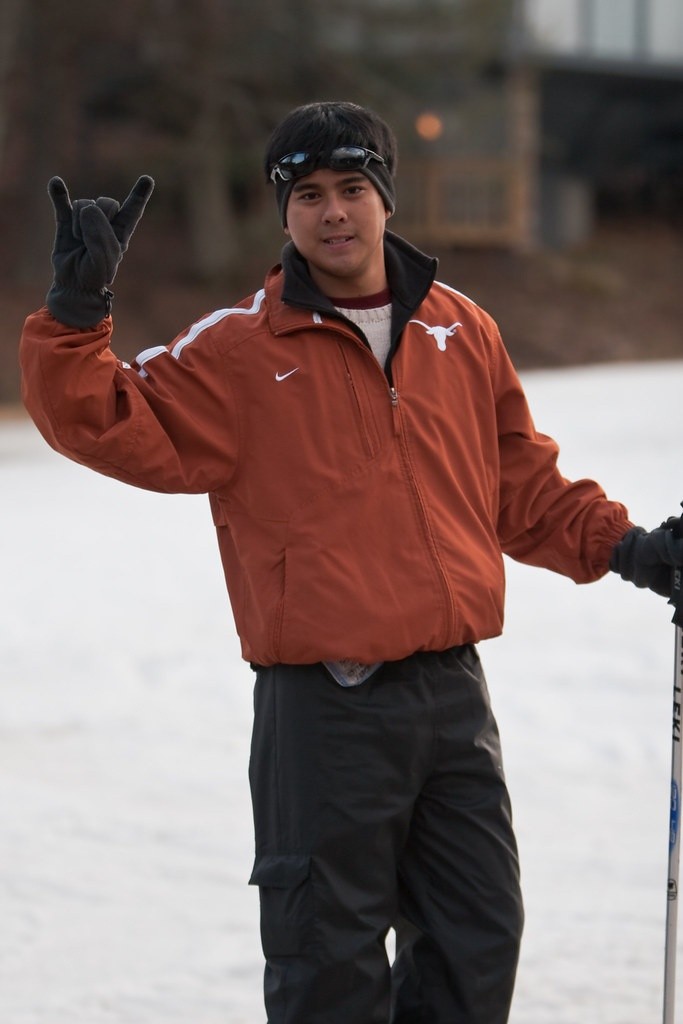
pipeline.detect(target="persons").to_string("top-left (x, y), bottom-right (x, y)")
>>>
top-left (19, 96), bottom-right (683, 1024)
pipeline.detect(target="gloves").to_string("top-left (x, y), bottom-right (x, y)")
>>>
top-left (44, 175), bottom-right (154, 328)
top-left (611, 517), bottom-right (683, 596)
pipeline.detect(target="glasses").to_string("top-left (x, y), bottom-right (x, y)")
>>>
top-left (269, 146), bottom-right (387, 186)
